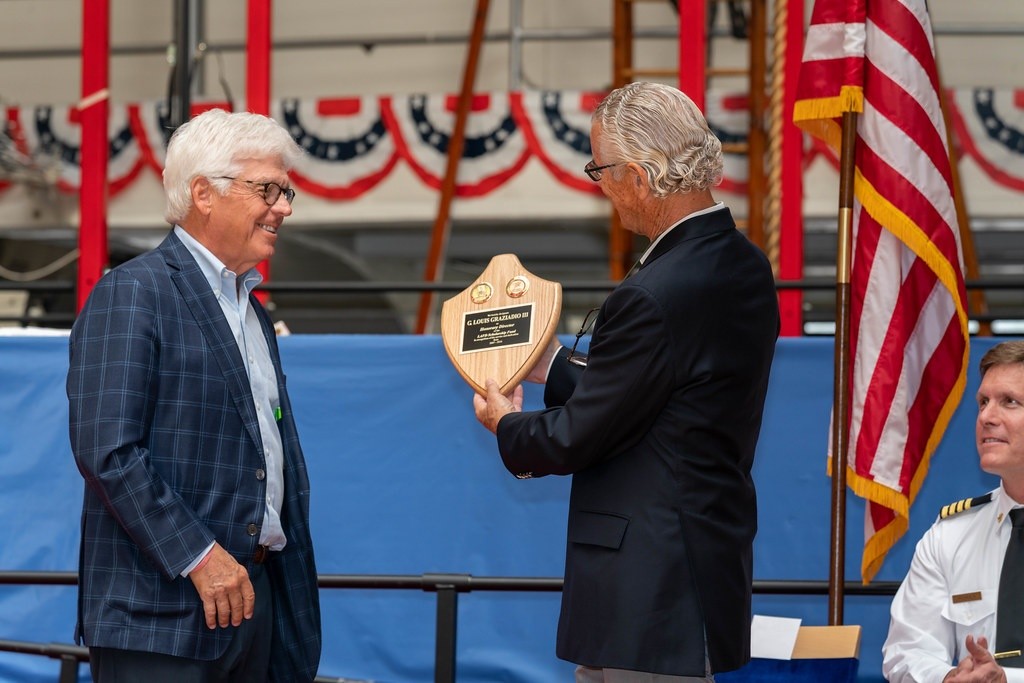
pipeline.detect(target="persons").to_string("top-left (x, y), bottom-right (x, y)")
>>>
top-left (64, 107), bottom-right (323, 683)
top-left (472, 83), bottom-right (766, 683)
top-left (881, 340), bottom-right (1024, 683)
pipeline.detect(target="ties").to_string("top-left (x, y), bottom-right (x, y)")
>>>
top-left (994, 508), bottom-right (1024, 670)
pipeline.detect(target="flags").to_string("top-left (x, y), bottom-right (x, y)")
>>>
top-left (792, 0), bottom-right (967, 585)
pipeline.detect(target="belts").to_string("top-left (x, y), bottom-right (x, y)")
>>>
top-left (254, 545), bottom-right (273, 564)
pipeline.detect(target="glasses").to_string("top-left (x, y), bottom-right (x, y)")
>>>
top-left (213, 175), bottom-right (295, 206)
top-left (566, 303), bottom-right (602, 368)
top-left (584, 160), bottom-right (616, 182)
